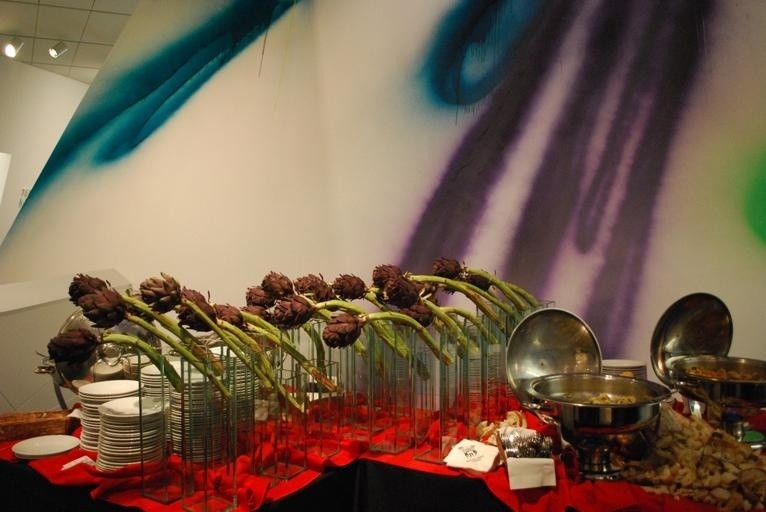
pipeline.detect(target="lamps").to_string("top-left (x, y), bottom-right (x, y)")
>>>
top-left (4, 35), bottom-right (69, 59)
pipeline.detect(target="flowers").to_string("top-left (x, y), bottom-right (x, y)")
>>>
top-left (21, 247), bottom-right (555, 511)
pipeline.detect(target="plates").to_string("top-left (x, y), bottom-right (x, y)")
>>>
top-left (77, 346), bottom-right (258, 472)
top-left (10, 435), bottom-right (80, 460)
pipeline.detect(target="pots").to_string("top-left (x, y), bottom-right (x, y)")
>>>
top-left (519, 373), bottom-right (679, 483)
top-left (664, 353), bottom-right (765, 445)
